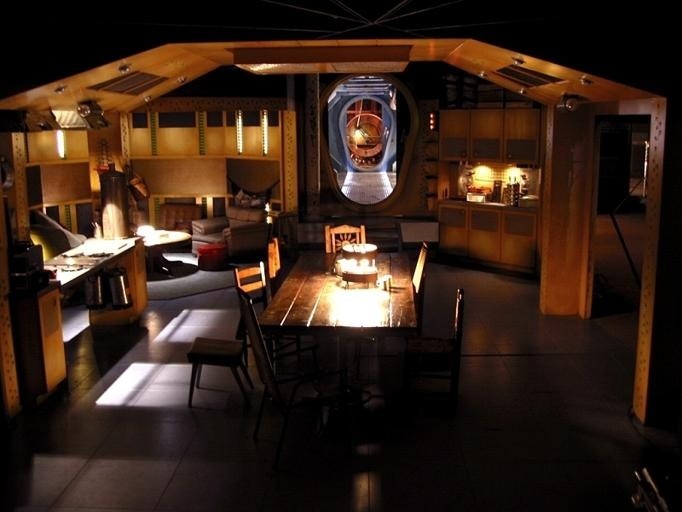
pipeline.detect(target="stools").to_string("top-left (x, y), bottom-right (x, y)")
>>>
top-left (197, 243), bottom-right (227, 271)
top-left (186, 337), bottom-right (254, 409)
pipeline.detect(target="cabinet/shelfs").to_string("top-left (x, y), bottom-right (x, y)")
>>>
top-left (438, 107), bottom-right (542, 275)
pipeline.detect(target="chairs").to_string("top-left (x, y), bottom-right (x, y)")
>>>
top-left (158, 203), bottom-right (202, 233)
top-left (190, 206), bottom-right (269, 258)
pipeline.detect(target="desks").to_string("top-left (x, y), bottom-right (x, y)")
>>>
top-left (16, 236), bottom-right (150, 408)
top-left (135, 230), bottom-right (192, 257)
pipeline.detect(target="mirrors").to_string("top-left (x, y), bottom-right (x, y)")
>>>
top-left (320, 73), bottom-right (420, 214)
